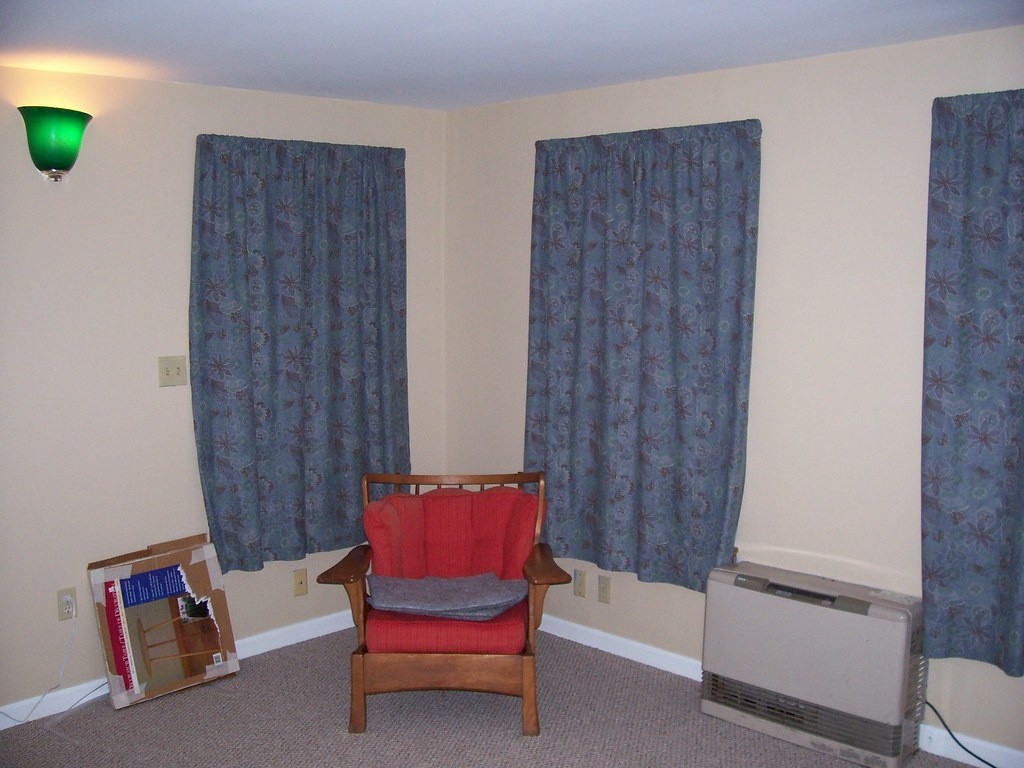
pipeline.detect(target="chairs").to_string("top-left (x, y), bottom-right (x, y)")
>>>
top-left (317, 471), bottom-right (571, 736)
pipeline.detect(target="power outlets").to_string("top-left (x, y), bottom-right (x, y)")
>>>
top-left (598, 575), bottom-right (611, 604)
top-left (158, 355), bottom-right (187, 387)
top-left (57, 587), bottom-right (78, 620)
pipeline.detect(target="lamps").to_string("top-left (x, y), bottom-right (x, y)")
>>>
top-left (17, 106), bottom-right (92, 183)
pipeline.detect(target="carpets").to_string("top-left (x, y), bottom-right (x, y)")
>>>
top-left (0, 624), bottom-right (979, 768)
